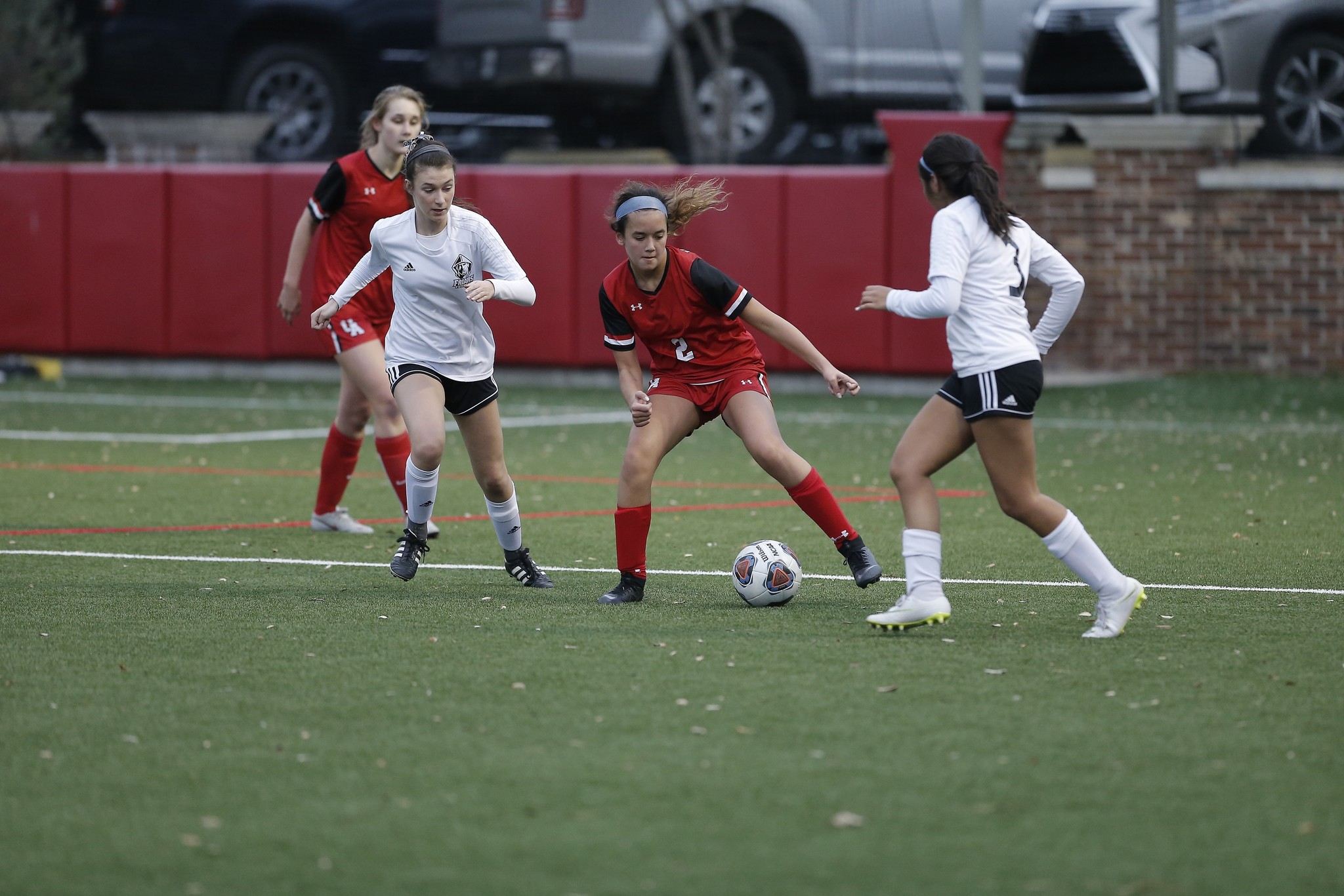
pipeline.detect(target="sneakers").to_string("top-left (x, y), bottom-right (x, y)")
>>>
top-left (390, 536), bottom-right (428, 582)
top-left (311, 508), bottom-right (375, 535)
top-left (1081, 577), bottom-right (1147, 638)
top-left (598, 572), bottom-right (644, 605)
top-left (866, 595), bottom-right (951, 630)
top-left (504, 548), bottom-right (555, 588)
top-left (843, 539), bottom-right (883, 588)
top-left (404, 514), bottom-right (439, 536)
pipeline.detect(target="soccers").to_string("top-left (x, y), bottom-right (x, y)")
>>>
top-left (735, 539), bottom-right (802, 605)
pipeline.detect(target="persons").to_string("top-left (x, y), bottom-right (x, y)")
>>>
top-left (599, 176), bottom-right (882, 604)
top-left (277, 84), bottom-right (443, 542)
top-left (855, 128), bottom-right (1145, 638)
top-left (309, 130), bottom-right (557, 589)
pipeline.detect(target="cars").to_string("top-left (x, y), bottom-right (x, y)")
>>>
top-left (59, 0), bottom-right (553, 161)
top-left (1013, 0), bottom-right (1344, 154)
top-left (423, 0), bottom-right (1223, 164)
